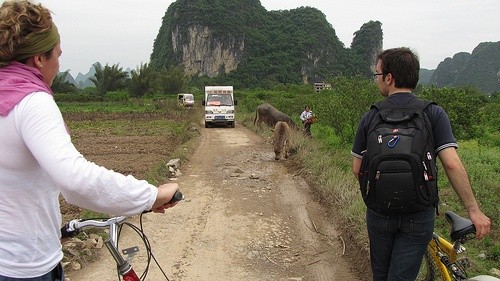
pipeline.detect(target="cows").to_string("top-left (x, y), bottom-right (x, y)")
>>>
top-left (254, 102), bottom-right (300, 133)
top-left (271, 121), bottom-right (290, 160)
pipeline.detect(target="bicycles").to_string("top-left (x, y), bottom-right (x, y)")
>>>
top-left (60, 188), bottom-right (185, 281)
top-left (415, 211), bottom-right (499, 281)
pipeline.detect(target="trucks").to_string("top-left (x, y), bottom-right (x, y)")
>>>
top-left (202, 86), bottom-right (237, 128)
top-left (177, 94), bottom-right (195, 108)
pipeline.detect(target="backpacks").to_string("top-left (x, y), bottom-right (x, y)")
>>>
top-left (358, 100), bottom-right (440, 214)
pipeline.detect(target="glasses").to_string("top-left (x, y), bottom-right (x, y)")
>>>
top-left (373, 72), bottom-right (382, 79)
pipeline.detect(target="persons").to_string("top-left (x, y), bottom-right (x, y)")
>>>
top-left (300, 105), bottom-right (317, 139)
top-left (351, 48), bottom-right (491, 281)
top-left (0, 0), bottom-right (182, 281)
